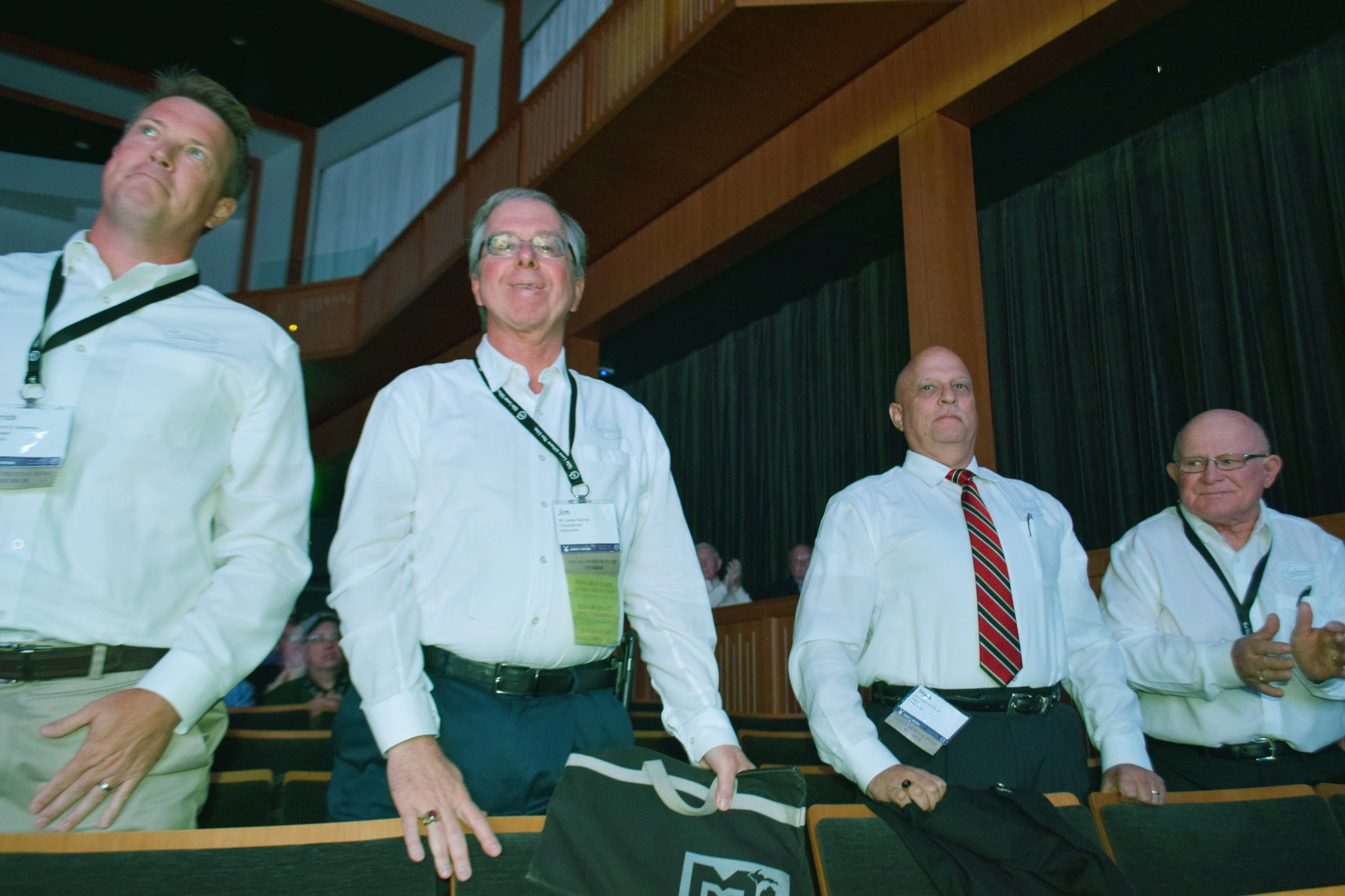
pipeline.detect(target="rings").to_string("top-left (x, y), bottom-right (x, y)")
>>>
top-left (421, 811), bottom-right (441, 825)
top-left (901, 778), bottom-right (914, 793)
top-left (1152, 789), bottom-right (1161, 796)
top-left (97, 780), bottom-right (113, 793)
top-left (1333, 651), bottom-right (1340, 663)
top-left (1259, 670), bottom-right (1265, 683)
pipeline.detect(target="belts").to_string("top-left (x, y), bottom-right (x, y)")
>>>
top-left (868, 686), bottom-right (1060, 716)
top-left (0, 644), bottom-right (170, 684)
top-left (421, 645), bottom-right (616, 696)
top-left (1143, 733), bottom-right (1335, 762)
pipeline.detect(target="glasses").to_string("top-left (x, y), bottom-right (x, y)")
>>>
top-left (478, 234), bottom-right (577, 267)
top-left (304, 632), bottom-right (337, 643)
top-left (1176, 453), bottom-right (1267, 473)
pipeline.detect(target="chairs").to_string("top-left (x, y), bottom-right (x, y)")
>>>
top-left (0, 713), bottom-right (1345, 896)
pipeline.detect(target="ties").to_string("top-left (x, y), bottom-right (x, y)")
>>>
top-left (945, 469), bottom-right (1023, 688)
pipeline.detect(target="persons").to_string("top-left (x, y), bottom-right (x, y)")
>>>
top-left (329, 187), bottom-right (756, 881)
top-left (0, 69), bottom-right (314, 833)
top-left (789, 347), bottom-right (1170, 805)
top-left (221, 541), bottom-right (808, 713)
top-left (1092, 409), bottom-right (1345, 787)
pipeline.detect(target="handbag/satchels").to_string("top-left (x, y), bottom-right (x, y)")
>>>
top-left (525, 745), bottom-right (814, 896)
